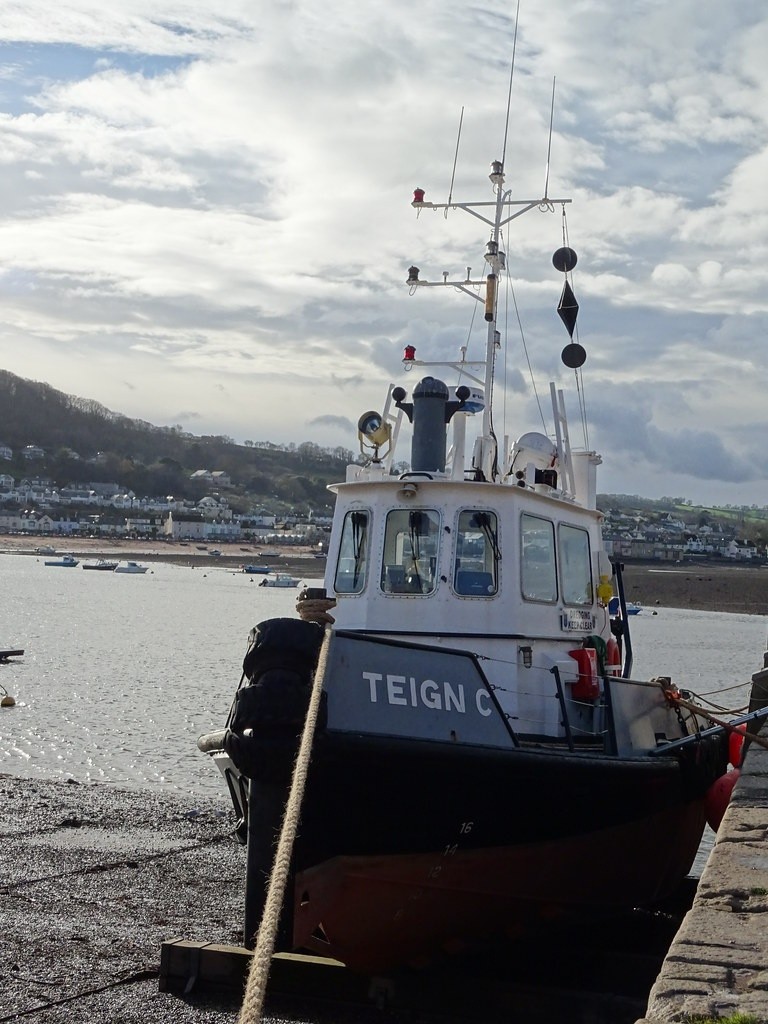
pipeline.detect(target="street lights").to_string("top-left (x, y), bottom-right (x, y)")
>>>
top-left (325, 504), bottom-right (334, 517)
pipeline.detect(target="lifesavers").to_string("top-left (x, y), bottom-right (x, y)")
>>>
top-left (606, 638), bottom-right (622, 677)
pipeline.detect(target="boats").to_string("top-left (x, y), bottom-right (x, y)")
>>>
top-left (314, 552), bottom-right (327, 558)
top-left (618, 602), bottom-right (641, 614)
top-left (197, 2), bottom-right (735, 1024)
top-left (196, 545), bottom-right (208, 550)
top-left (209, 549), bottom-right (222, 555)
top-left (115, 560), bottom-right (149, 573)
top-left (45, 552), bottom-right (80, 567)
top-left (258, 552), bottom-right (280, 557)
top-left (244, 562), bottom-right (274, 574)
top-left (262, 574), bottom-right (302, 587)
top-left (82, 551), bottom-right (118, 570)
